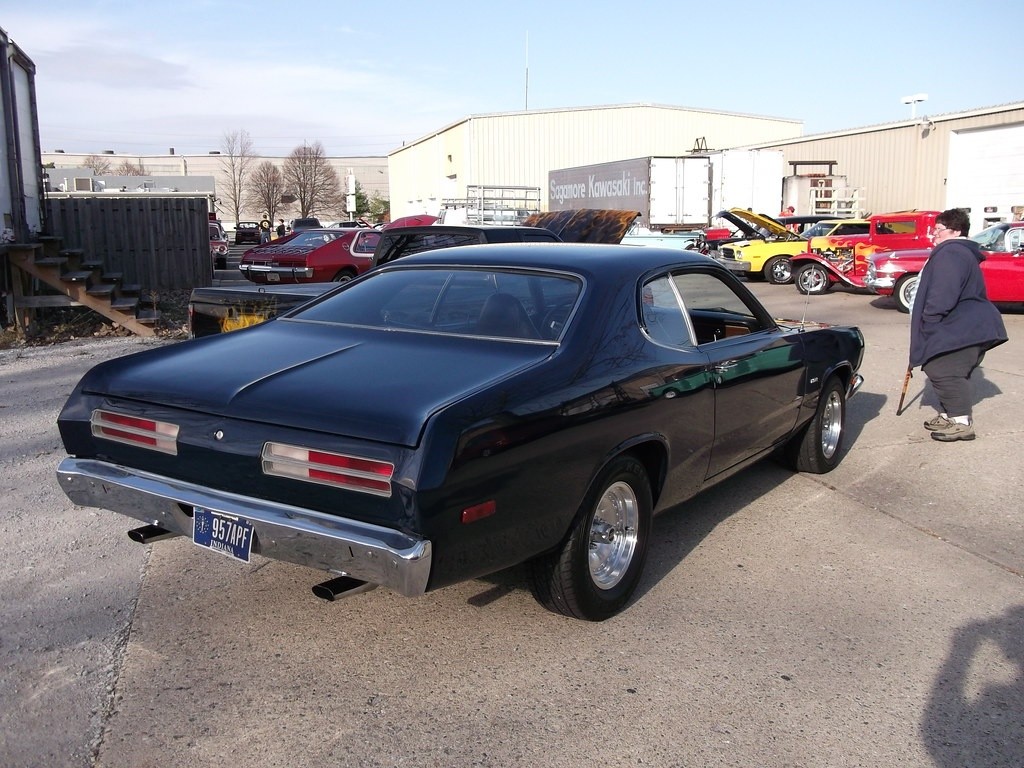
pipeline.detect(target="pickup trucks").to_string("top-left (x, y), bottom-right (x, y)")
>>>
top-left (185, 223), bottom-right (654, 348)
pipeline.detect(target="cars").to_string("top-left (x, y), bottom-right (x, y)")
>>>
top-left (52, 240), bottom-right (870, 625)
top-left (861, 220), bottom-right (1024, 318)
top-left (680, 206), bottom-right (915, 285)
top-left (328, 219), bottom-right (387, 246)
top-left (286, 217), bottom-right (325, 235)
top-left (784, 208), bottom-right (943, 297)
top-left (209, 220), bottom-right (230, 280)
top-left (234, 222), bottom-right (263, 244)
top-left (237, 214), bottom-right (443, 285)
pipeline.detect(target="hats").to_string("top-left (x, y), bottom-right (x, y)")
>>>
top-left (786, 206), bottom-right (795, 213)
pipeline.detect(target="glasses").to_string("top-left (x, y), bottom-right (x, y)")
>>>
top-left (932, 227), bottom-right (948, 233)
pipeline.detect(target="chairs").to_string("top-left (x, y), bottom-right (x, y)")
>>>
top-left (478, 293), bottom-right (543, 340)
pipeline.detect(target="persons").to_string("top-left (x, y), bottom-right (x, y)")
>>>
top-left (746, 208), bottom-right (753, 228)
top-left (907, 209), bottom-right (1008, 442)
top-left (275, 219), bottom-right (285, 238)
top-left (779, 206), bottom-right (798, 233)
top-left (286, 225), bottom-right (291, 231)
top-left (259, 214), bottom-right (271, 245)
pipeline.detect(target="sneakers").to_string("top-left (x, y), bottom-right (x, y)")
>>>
top-left (923, 413), bottom-right (956, 431)
top-left (930, 419), bottom-right (976, 442)
